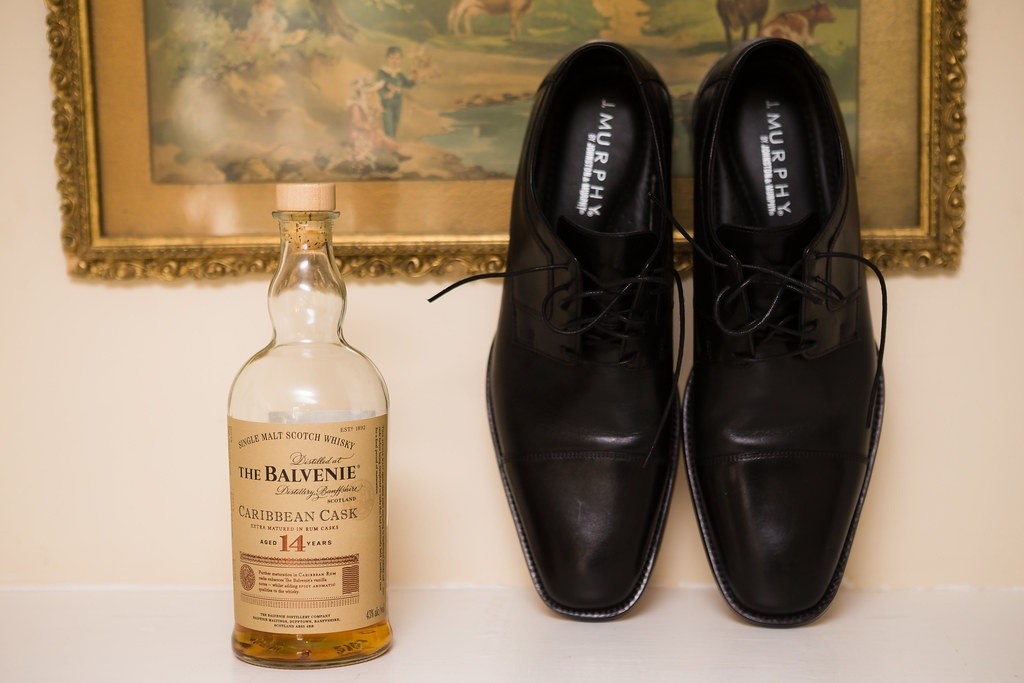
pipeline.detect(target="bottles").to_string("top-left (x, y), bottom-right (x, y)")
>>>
top-left (221, 183), bottom-right (396, 668)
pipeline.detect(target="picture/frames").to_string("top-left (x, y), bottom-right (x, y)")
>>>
top-left (44, 0), bottom-right (969, 277)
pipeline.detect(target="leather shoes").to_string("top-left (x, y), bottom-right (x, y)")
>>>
top-left (692, 36), bottom-right (886, 629)
top-left (427, 42), bottom-right (682, 619)
